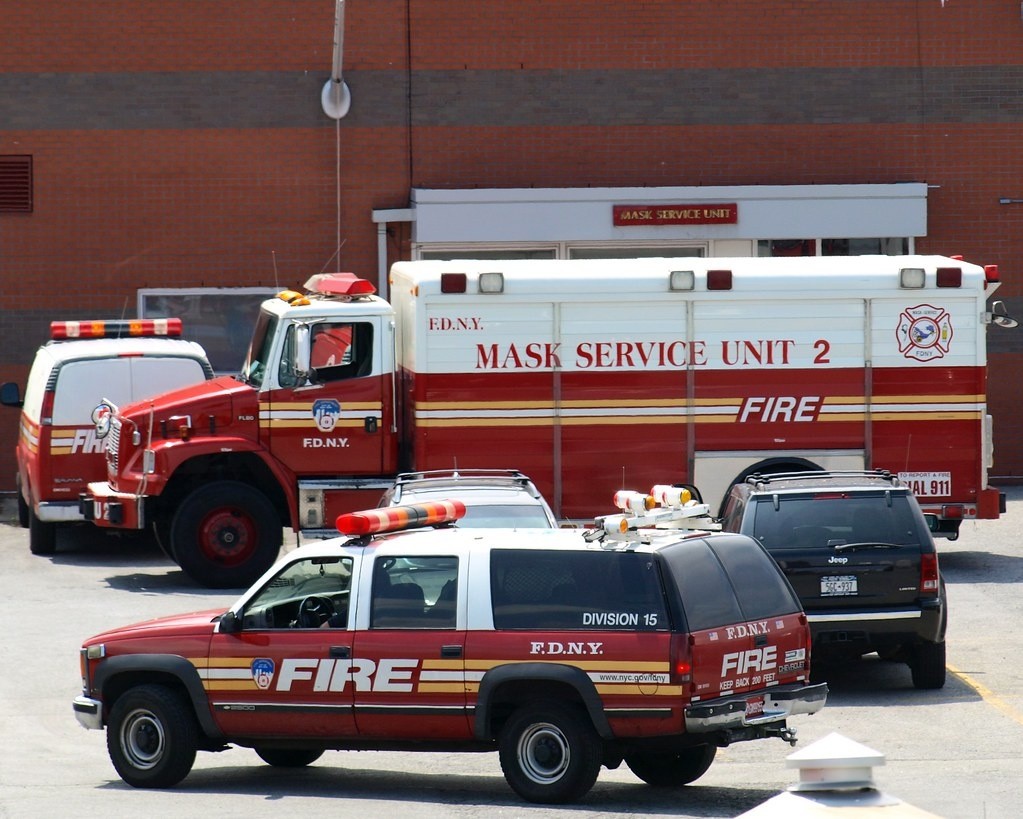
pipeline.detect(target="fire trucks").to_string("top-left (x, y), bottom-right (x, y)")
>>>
top-left (75, 257), bottom-right (1018, 593)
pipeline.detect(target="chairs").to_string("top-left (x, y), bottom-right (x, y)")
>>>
top-left (852, 508), bottom-right (890, 539)
top-left (372, 577), bottom-right (458, 627)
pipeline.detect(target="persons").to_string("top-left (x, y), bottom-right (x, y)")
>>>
top-left (320, 572), bottom-right (391, 628)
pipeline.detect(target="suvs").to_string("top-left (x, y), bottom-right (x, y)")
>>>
top-left (377, 470), bottom-right (563, 605)
top-left (719, 469), bottom-right (948, 690)
top-left (69, 481), bottom-right (830, 805)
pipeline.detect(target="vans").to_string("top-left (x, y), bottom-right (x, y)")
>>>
top-left (0, 317), bottom-right (217, 557)
